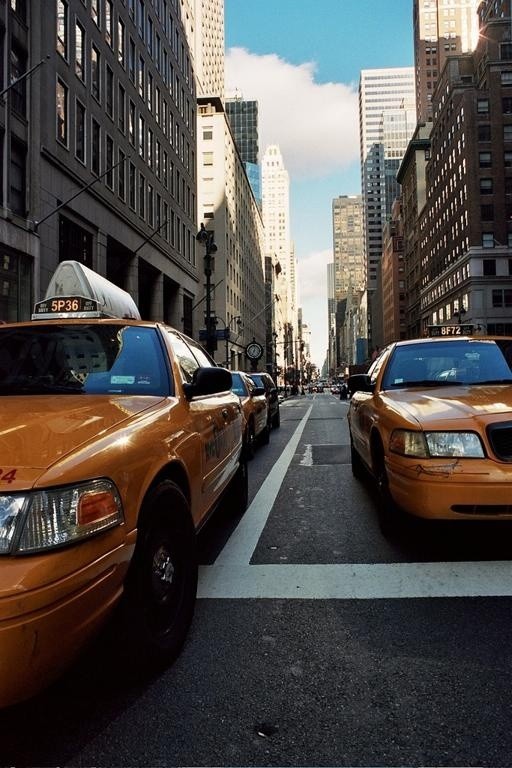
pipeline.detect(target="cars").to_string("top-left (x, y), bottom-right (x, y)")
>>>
top-left (0, 261), bottom-right (249, 707)
top-left (228, 370), bottom-right (272, 461)
top-left (308, 378), bottom-right (328, 394)
top-left (248, 372), bottom-right (281, 430)
top-left (347, 324), bottom-right (511, 521)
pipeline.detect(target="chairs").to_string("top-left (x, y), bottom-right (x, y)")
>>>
top-left (395, 361), bottom-right (428, 383)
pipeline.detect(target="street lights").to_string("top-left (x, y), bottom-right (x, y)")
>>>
top-left (196, 223), bottom-right (217, 356)
top-left (273, 340), bottom-right (305, 383)
